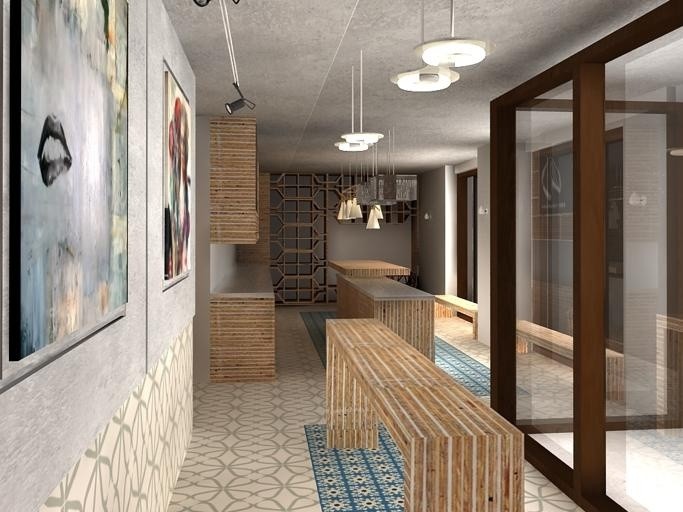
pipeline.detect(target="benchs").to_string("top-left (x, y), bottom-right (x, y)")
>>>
top-left (433, 294), bottom-right (477, 340)
top-left (515, 320), bottom-right (625, 402)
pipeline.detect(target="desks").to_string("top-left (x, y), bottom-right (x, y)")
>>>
top-left (325, 318), bottom-right (525, 511)
top-left (208, 261), bottom-right (277, 384)
top-left (329, 260), bottom-right (410, 279)
top-left (335, 276), bottom-right (435, 365)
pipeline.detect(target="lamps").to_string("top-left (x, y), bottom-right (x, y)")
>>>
top-left (332, 0), bottom-right (499, 231)
top-left (219, 0), bottom-right (256, 116)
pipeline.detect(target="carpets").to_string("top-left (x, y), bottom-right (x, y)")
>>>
top-left (304, 421), bottom-right (407, 512)
top-left (299, 311), bottom-right (491, 398)
